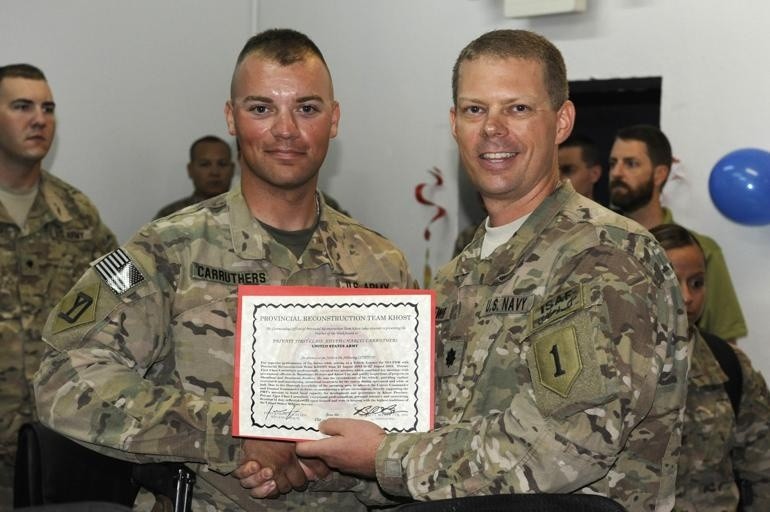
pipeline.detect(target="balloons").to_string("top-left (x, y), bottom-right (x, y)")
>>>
top-left (707, 147), bottom-right (769, 228)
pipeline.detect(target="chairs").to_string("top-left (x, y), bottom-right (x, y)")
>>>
top-left (16, 423), bottom-right (195, 510)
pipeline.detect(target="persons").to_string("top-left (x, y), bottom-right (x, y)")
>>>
top-left (0, 62), bottom-right (125, 510)
top-left (29, 27), bottom-right (416, 512)
top-left (227, 28), bottom-right (698, 512)
top-left (147, 132), bottom-right (238, 221)
top-left (557, 132), bottom-right (606, 207)
top-left (599, 123), bottom-right (751, 354)
top-left (638, 221), bottom-right (769, 512)
